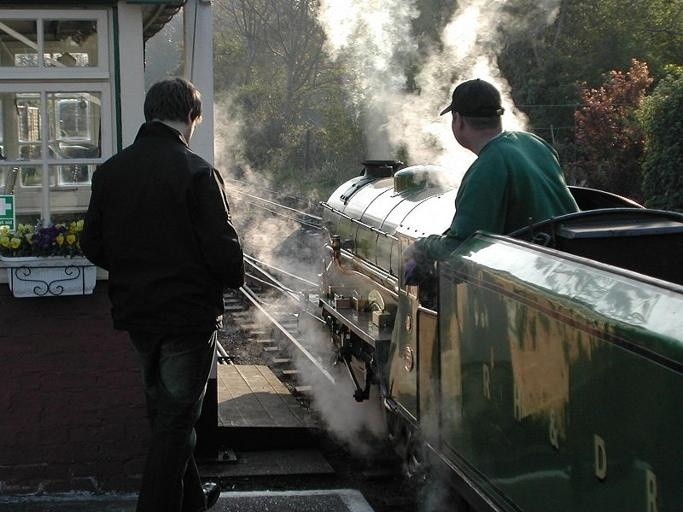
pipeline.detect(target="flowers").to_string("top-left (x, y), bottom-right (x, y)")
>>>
top-left (0, 217), bottom-right (90, 260)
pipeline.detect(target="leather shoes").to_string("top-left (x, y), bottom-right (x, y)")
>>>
top-left (182, 480), bottom-right (221, 512)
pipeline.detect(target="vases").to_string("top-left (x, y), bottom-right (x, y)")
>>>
top-left (0, 251), bottom-right (101, 298)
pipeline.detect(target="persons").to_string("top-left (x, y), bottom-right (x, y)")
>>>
top-left (399, 77), bottom-right (579, 278)
top-left (78, 79), bottom-right (244, 512)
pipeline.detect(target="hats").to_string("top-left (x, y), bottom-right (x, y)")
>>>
top-left (440, 78), bottom-right (504, 117)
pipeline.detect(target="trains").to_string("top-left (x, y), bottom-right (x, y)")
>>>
top-left (311, 155), bottom-right (682, 512)
